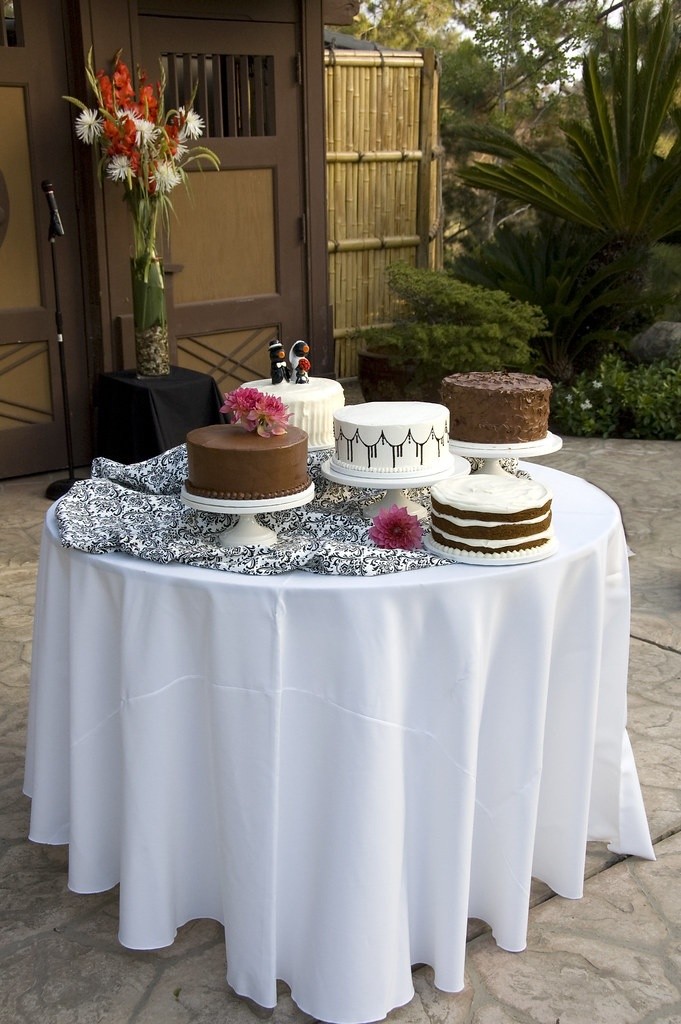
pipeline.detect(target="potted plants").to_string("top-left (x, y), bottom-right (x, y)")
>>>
top-left (343, 259), bottom-right (554, 404)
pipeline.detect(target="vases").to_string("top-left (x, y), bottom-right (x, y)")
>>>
top-left (131, 252), bottom-right (171, 379)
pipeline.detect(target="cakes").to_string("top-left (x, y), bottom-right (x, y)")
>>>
top-left (184, 387), bottom-right (312, 500)
top-left (331, 401), bottom-right (451, 472)
top-left (427, 472), bottom-right (552, 559)
top-left (239, 338), bottom-right (345, 447)
top-left (439, 370), bottom-right (553, 444)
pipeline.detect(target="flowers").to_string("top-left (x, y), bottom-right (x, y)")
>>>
top-left (369, 505), bottom-right (423, 550)
top-left (61, 47), bottom-right (221, 338)
top-left (218, 388), bottom-right (294, 437)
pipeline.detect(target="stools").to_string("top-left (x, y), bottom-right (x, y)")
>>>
top-left (94, 365), bottom-right (232, 467)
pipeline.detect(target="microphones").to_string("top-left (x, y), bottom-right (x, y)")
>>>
top-left (41, 179), bottom-right (65, 236)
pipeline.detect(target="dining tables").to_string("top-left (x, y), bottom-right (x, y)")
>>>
top-left (22, 441), bottom-right (657, 1024)
